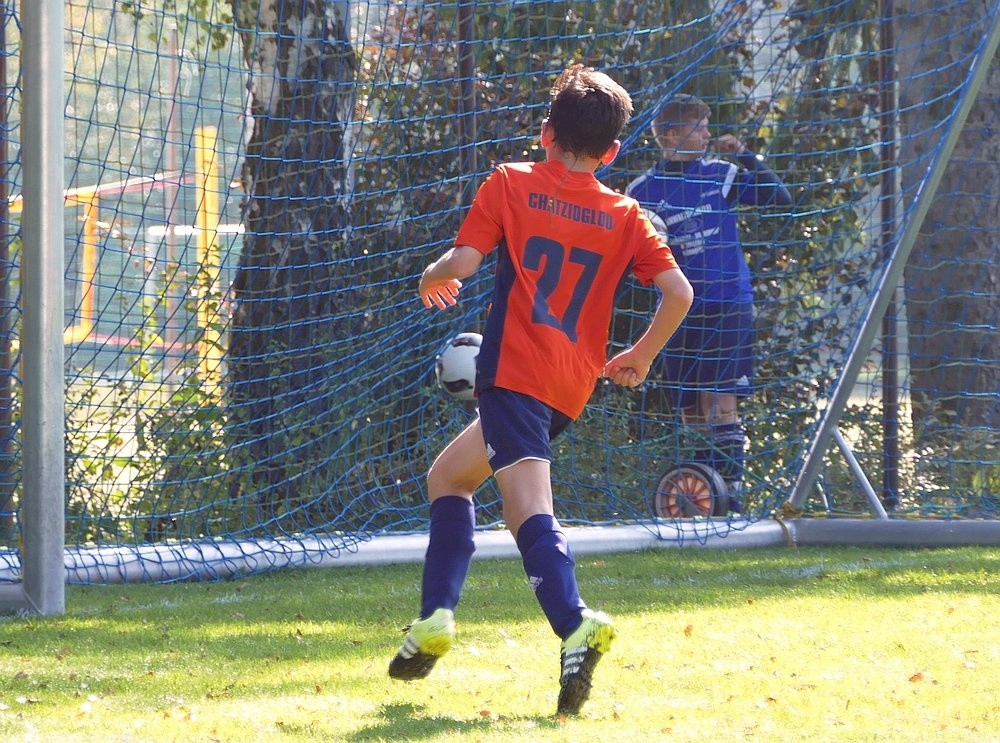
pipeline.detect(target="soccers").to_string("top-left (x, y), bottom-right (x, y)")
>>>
top-left (435, 332), bottom-right (484, 401)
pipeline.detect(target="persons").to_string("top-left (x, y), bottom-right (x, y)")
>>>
top-left (383, 60), bottom-right (698, 720)
top-left (623, 94), bottom-right (798, 517)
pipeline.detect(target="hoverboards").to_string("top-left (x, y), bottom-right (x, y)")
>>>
top-left (653, 442), bottom-right (757, 528)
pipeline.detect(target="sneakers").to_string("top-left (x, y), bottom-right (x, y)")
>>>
top-left (557, 609), bottom-right (620, 721)
top-left (388, 607), bottom-right (457, 681)
